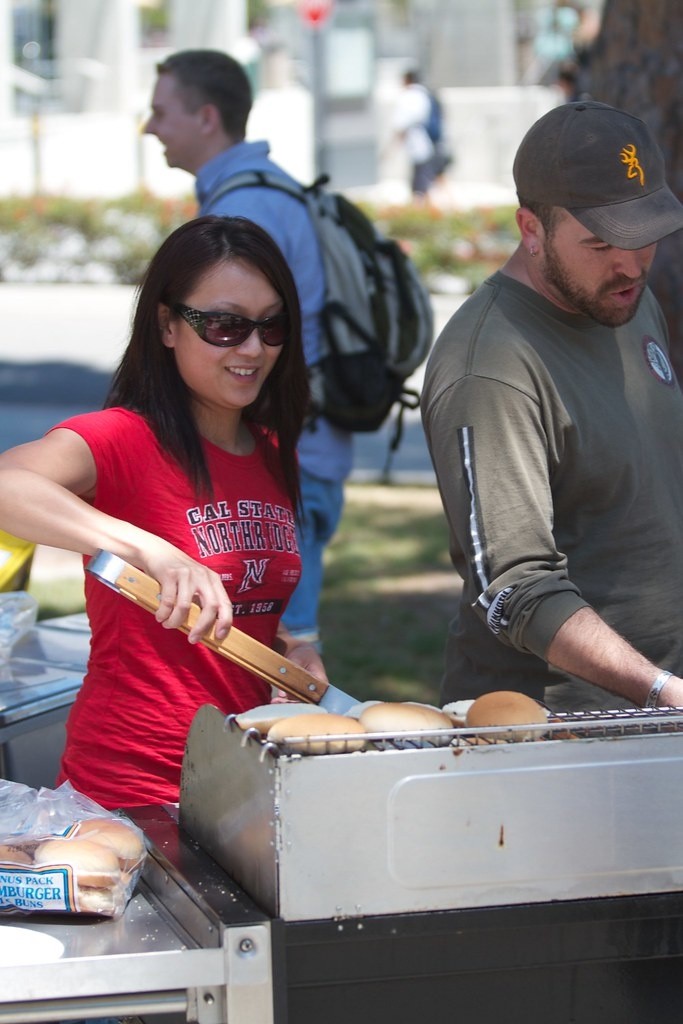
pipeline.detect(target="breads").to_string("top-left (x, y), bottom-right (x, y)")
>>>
top-left (0, 818), bottom-right (142, 914)
top-left (237, 690), bottom-right (550, 752)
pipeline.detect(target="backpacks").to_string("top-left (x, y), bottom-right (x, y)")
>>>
top-left (204, 169), bottom-right (434, 449)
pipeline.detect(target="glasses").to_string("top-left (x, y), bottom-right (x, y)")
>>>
top-left (169, 296), bottom-right (295, 347)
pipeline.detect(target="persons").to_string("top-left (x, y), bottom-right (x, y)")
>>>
top-left (142, 48), bottom-right (352, 652)
top-left (423, 101), bottom-right (683, 708)
top-left (392, 72), bottom-right (453, 208)
top-left (0, 214), bottom-right (331, 806)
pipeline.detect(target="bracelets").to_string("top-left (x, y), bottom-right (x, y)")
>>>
top-left (645, 671), bottom-right (674, 709)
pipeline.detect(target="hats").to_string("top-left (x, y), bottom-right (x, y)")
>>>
top-left (513, 101), bottom-right (683, 250)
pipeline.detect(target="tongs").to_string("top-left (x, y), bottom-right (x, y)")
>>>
top-left (86, 550), bottom-right (362, 719)
